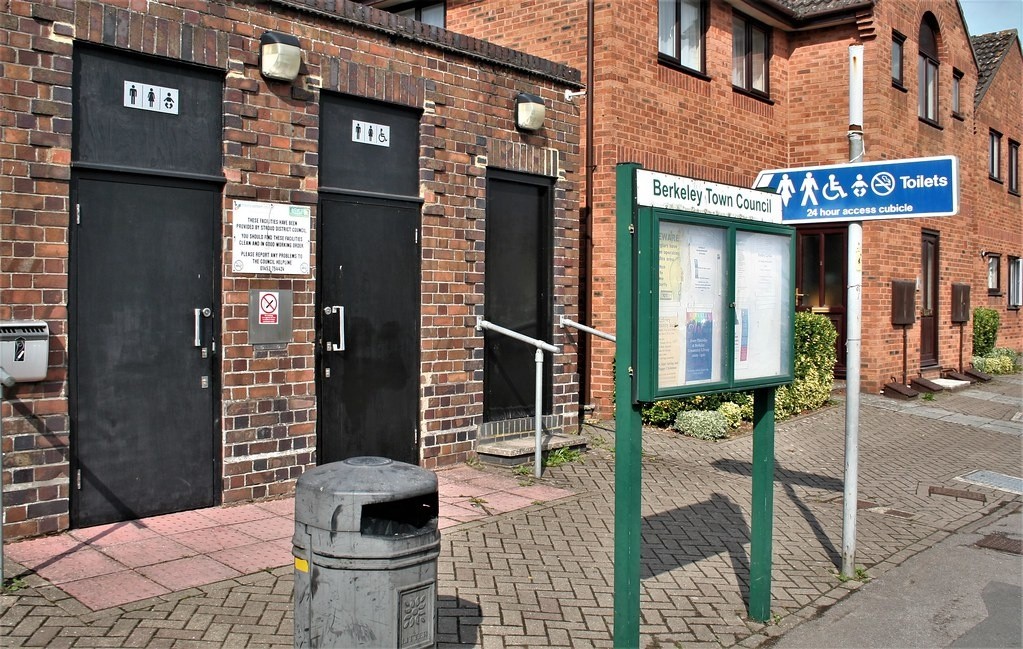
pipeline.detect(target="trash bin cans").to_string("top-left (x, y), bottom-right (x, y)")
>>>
top-left (292, 458), bottom-right (441, 649)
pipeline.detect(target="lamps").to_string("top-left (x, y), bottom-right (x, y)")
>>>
top-left (258, 31), bottom-right (302, 81)
top-left (514, 93), bottom-right (545, 132)
